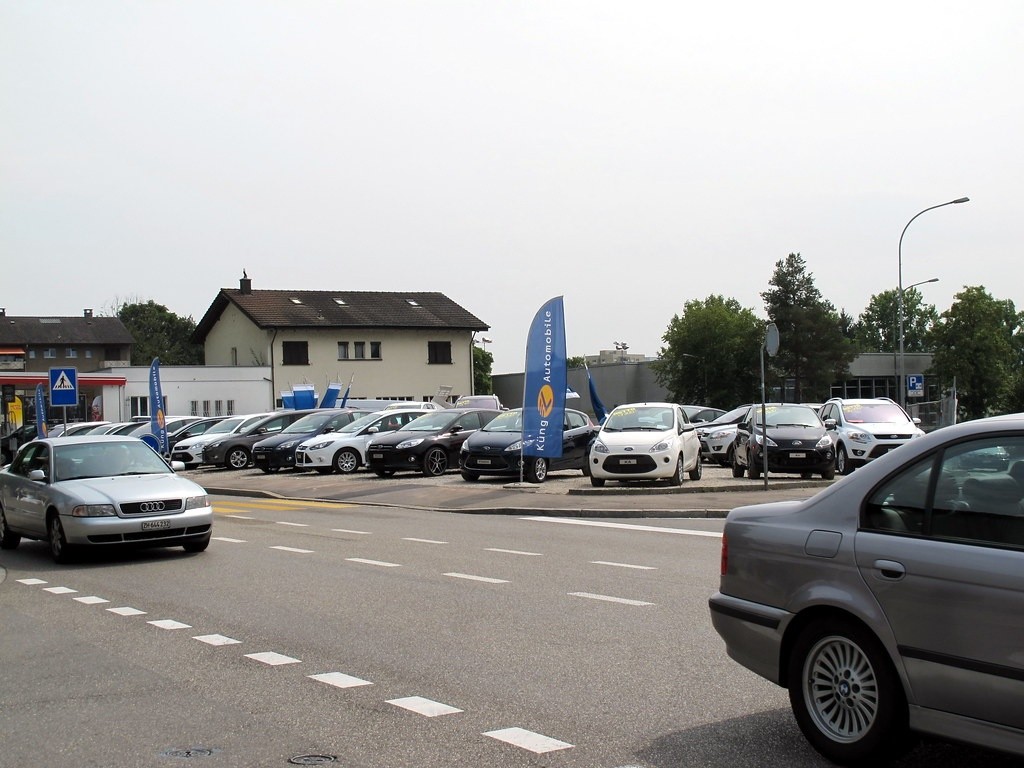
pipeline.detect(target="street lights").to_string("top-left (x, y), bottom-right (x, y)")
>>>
top-left (893, 278), bottom-right (939, 405)
top-left (898, 197), bottom-right (972, 411)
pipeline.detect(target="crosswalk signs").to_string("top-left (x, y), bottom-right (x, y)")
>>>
top-left (53, 370), bottom-right (75, 391)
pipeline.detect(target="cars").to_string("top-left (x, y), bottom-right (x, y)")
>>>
top-left (252, 411), bottom-right (373, 474)
top-left (171, 414), bottom-right (277, 469)
top-left (0, 415), bottom-right (228, 467)
top-left (454, 395), bottom-right (504, 410)
top-left (734, 401), bottom-right (836, 480)
top-left (708, 413), bottom-right (1023, 765)
top-left (588, 402), bottom-right (701, 487)
top-left (460, 408), bottom-right (597, 483)
top-left (1, 435), bottom-right (213, 562)
top-left (294, 410), bottom-right (436, 473)
top-left (366, 408), bottom-right (509, 478)
top-left (204, 412), bottom-right (310, 469)
top-left (676, 401), bottom-right (751, 467)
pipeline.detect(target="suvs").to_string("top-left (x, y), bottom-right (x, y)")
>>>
top-left (820, 397), bottom-right (928, 475)
